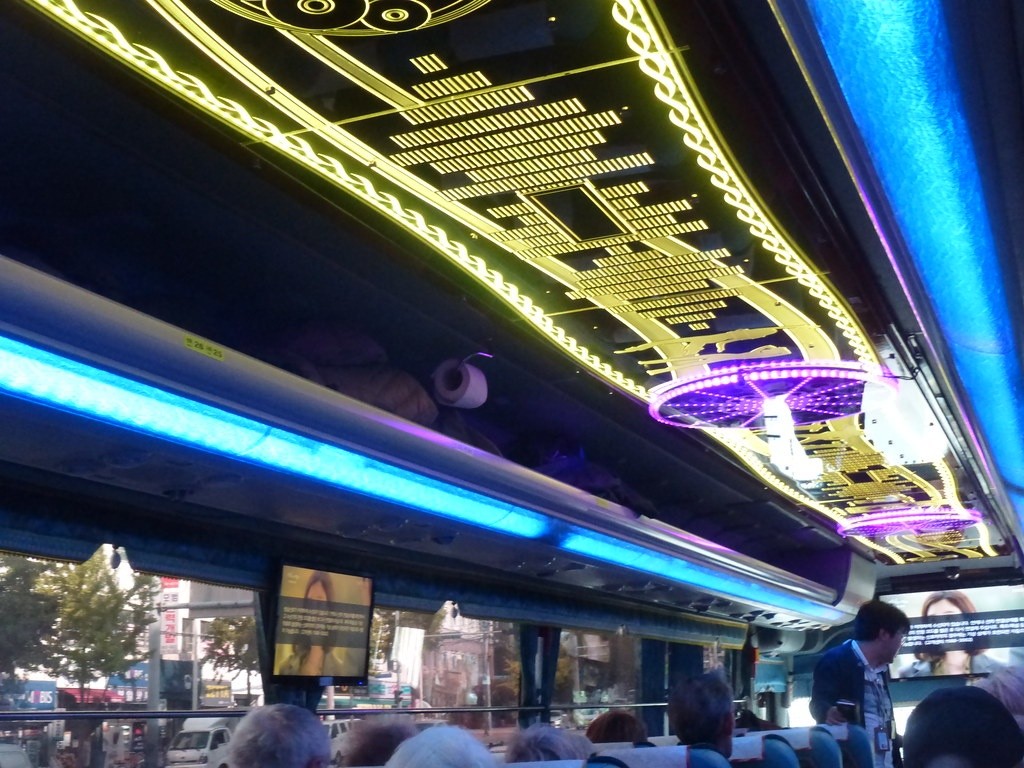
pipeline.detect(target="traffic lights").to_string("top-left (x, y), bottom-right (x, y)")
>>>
top-left (131, 721), bottom-right (149, 752)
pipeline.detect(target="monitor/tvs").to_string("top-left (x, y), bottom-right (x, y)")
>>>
top-left (265, 557), bottom-right (376, 685)
top-left (875, 579), bottom-right (1024, 681)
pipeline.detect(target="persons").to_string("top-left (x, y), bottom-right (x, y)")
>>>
top-left (278, 571), bottom-right (357, 676)
top-left (810, 600), bottom-right (911, 767)
top-left (896, 590), bottom-right (1006, 678)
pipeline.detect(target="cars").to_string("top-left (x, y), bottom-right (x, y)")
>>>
top-left (550, 710), bottom-right (570, 730)
top-left (577, 709), bottom-right (600, 729)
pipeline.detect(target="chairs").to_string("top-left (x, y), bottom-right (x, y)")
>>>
top-left (505, 722), bottom-right (880, 767)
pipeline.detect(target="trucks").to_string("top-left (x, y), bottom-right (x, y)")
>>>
top-left (165, 717), bottom-right (240, 768)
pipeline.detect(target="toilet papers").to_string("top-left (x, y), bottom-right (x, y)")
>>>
top-left (432, 359), bottom-right (489, 411)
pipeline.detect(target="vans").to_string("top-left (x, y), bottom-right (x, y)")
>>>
top-left (320, 719), bottom-right (363, 764)
